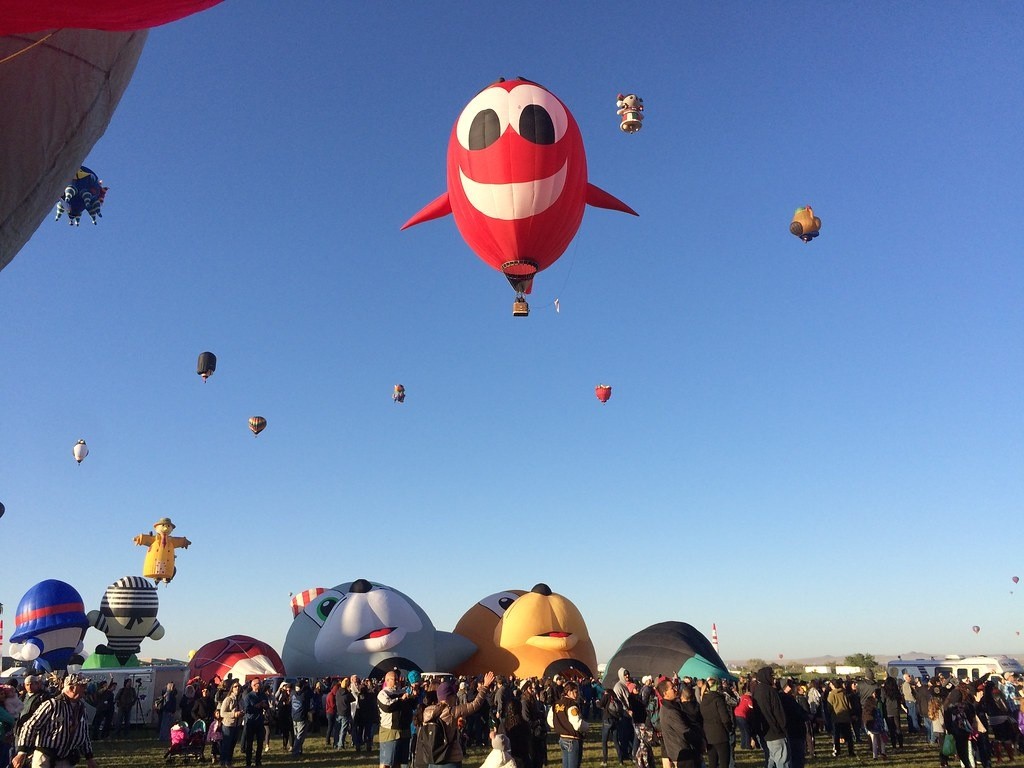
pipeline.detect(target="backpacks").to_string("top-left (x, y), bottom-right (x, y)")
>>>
top-left (154, 699), bottom-right (163, 714)
top-left (414, 703), bottom-right (450, 768)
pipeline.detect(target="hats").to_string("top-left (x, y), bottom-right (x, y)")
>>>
top-left (641, 675), bottom-right (653, 683)
top-left (64, 673), bottom-right (86, 686)
top-left (625, 681), bottom-right (636, 693)
top-left (24, 675), bottom-right (37, 683)
top-left (408, 670), bottom-right (421, 684)
top-left (520, 679), bottom-right (531, 692)
top-left (280, 682), bottom-right (289, 688)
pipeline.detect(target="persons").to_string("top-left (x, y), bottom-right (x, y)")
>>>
top-left (0, 672), bottom-right (136, 768)
top-left (155, 666), bottom-right (1024, 768)
top-left (515, 297), bottom-right (524, 302)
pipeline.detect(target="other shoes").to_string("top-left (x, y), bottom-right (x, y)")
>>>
top-left (90, 731), bottom-right (130, 739)
top-left (741, 746), bottom-right (759, 749)
top-left (847, 752), bottom-right (858, 757)
top-left (873, 746), bottom-right (904, 760)
top-left (220, 763), bottom-right (264, 768)
top-left (602, 760), bottom-right (637, 767)
top-left (264, 747), bottom-right (269, 752)
top-left (833, 752), bottom-right (840, 757)
top-left (281, 746), bottom-right (301, 757)
top-left (326, 741), bottom-right (372, 754)
top-left (810, 756), bottom-right (818, 760)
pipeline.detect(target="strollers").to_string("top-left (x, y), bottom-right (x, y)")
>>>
top-left (165, 719), bottom-right (208, 765)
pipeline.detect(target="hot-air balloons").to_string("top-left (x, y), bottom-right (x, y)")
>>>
top-left (281, 578), bottom-right (478, 678)
top-left (196, 352), bottom-right (217, 385)
top-left (615, 90), bottom-right (646, 134)
top-left (0, 0), bottom-right (231, 273)
top-left (595, 384), bottom-right (612, 404)
top-left (1012, 576), bottom-right (1019, 585)
top-left (183, 634), bottom-right (286, 685)
top-left (248, 416), bottom-right (267, 441)
top-left (972, 625), bottom-right (980, 635)
top-left (9, 578), bottom-right (90, 688)
top-left (71, 439), bottom-right (90, 468)
top-left (392, 384), bottom-right (405, 405)
top-left (451, 583), bottom-right (599, 685)
top-left (790, 206), bottom-right (822, 244)
top-left (133, 518), bottom-right (192, 589)
top-left (81, 651), bottom-right (140, 668)
top-left (601, 621), bottom-right (739, 685)
top-left (86, 575), bottom-right (165, 668)
top-left (398, 74), bottom-right (642, 318)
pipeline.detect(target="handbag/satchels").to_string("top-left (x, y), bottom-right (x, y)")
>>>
top-left (942, 732), bottom-right (958, 756)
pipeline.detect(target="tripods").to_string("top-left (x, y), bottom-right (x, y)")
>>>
top-left (134, 682), bottom-right (147, 728)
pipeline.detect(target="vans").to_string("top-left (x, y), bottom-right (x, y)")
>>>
top-left (421, 672), bottom-right (453, 683)
top-left (887, 653), bottom-right (1024, 684)
